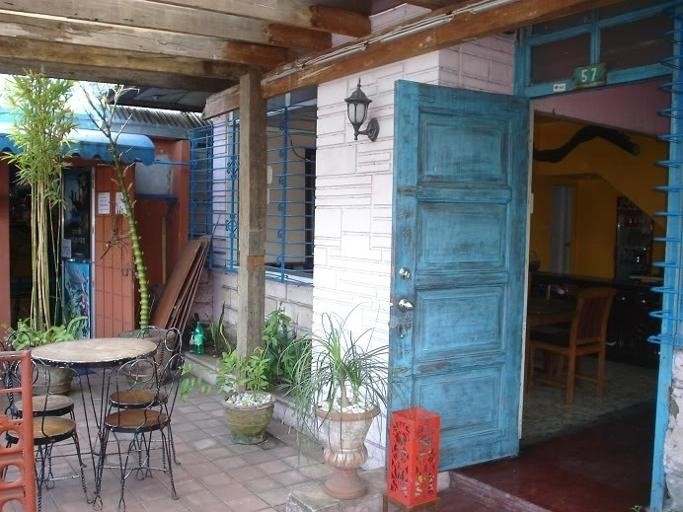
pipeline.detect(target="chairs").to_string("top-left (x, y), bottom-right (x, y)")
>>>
top-left (529, 287), bottom-right (615, 404)
top-left (0, 326), bottom-right (185, 512)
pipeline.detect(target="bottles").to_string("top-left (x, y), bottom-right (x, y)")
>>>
top-left (188, 311), bottom-right (204, 355)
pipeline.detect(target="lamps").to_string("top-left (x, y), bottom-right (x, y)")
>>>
top-left (341, 77), bottom-right (379, 143)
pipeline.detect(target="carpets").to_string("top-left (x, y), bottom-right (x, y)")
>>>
top-left (522, 350), bottom-right (657, 450)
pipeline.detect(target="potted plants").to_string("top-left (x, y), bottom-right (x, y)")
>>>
top-left (0, 316), bottom-right (87, 396)
top-left (216, 299), bottom-right (410, 499)
top-left (77, 82), bottom-right (178, 390)
